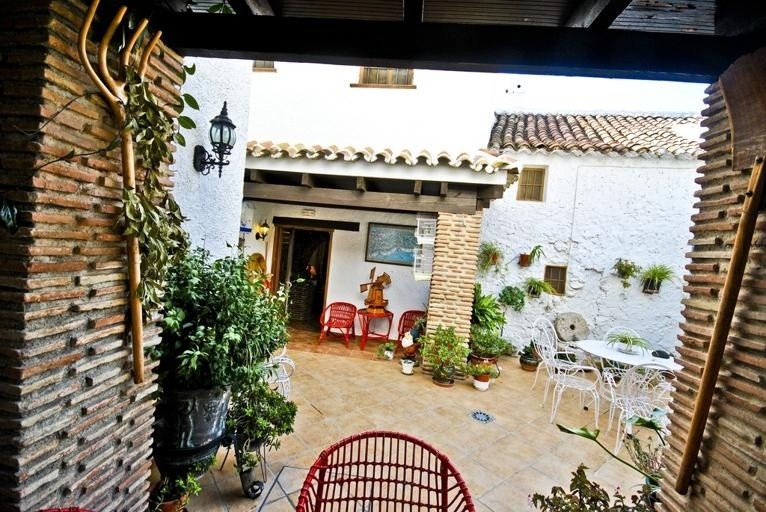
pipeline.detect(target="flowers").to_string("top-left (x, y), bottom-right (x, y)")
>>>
top-left (526, 449), bottom-right (658, 512)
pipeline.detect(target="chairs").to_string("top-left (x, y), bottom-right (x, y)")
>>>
top-left (530, 316), bottom-right (590, 405)
top-left (317, 299), bottom-right (358, 349)
top-left (295, 426), bottom-right (478, 512)
top-left (602, 359), bottom-right (677, 458)
top-left (541, 343), bottom-right (604, 434)
top-left (600, 325), bottom-right (653, 435)
top-left (255, 336), bottom-right (293, 398)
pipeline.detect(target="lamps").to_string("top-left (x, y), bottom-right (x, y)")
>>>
top-left (190, 100), bottom-right (240, 180)
top-left (255, 219), bottom-right (270, 242)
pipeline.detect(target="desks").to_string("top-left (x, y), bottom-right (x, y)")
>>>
top-left (356, 307), bottom-right (393, 351)
top-left (571, 329), bottom-right (684, 434)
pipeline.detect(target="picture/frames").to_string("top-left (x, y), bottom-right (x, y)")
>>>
top-left (365, 221), bottom-right (424, 268)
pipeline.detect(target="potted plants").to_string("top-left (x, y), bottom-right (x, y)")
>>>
top-left (605, 331), bottom-right (651, 356)
top-left (376, 238), bottom-right (558, 392)
top-left (642, 265), bottom-right (675, 296)
top-left (611, 256), bottom-right (638, 290)
top-left (152, 243), bottom-right (287, 483)
top-left (621, 439), bottom-right (661, 512)
top-left (219, 378), bottom-right (297, 455)
top-left (150, 470), bottom-right (201, 512)
